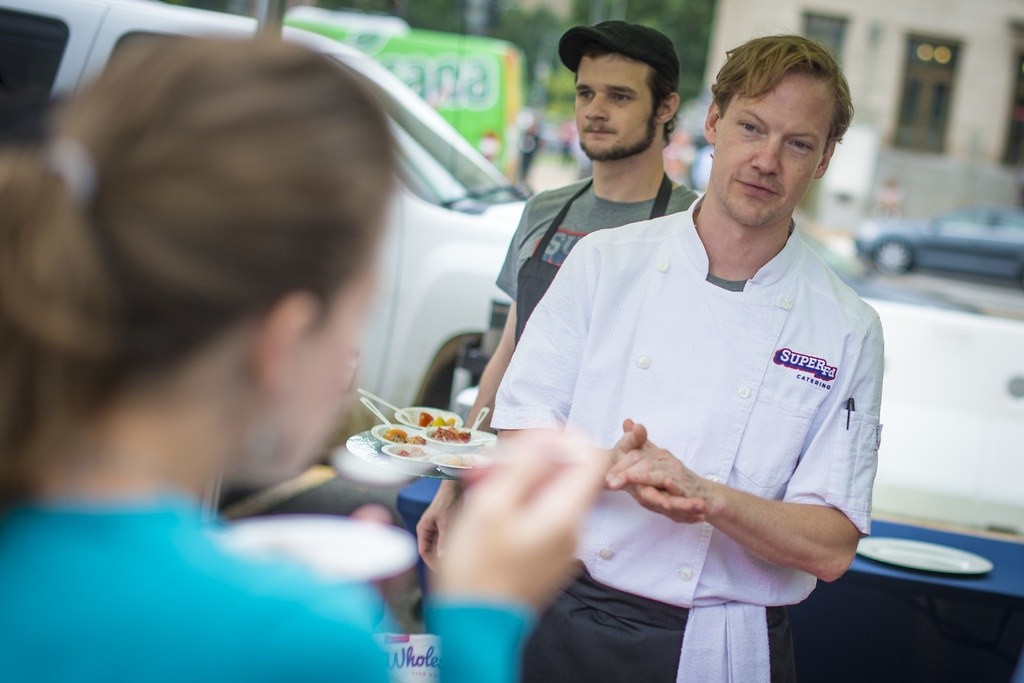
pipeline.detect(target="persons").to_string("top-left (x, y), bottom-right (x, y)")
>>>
top-left (415, 21), bottom-right (699, 569)
top-left (1, 36), bottom-right (607, 683)
top-left (491, 35), bottom-right (885, 683)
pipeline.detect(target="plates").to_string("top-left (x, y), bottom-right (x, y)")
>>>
top-left (221, 515), bottom-right (419, 585)
top-left (856, 535), bottom-right (994, 576)
top-left (331, 445), bottom-right (417, 488)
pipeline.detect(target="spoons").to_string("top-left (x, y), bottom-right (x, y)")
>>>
top-left (357, 389), bottom-right (417, 424)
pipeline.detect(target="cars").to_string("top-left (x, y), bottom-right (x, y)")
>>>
top-left (0, 0), bottom-right (529, 412)
top-left (855, 201), bottom-right (1024, 283)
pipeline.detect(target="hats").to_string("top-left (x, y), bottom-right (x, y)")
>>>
top-left (558, 20), bottom-right (680, 91)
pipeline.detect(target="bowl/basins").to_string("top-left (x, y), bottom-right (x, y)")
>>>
top-left (371, 424), bottom-right (428, 446)
top-left (429, 451), bottom-right (494, 476)
top-left (394, 407), bottom-right (464, 430)
top-left (422, 426), bottom-right (486, 452)
top-left (380, 444), bottom-right (434, 470)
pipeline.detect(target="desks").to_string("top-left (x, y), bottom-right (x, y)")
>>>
top-left (397, 471), bottom-right (1024, 683)
